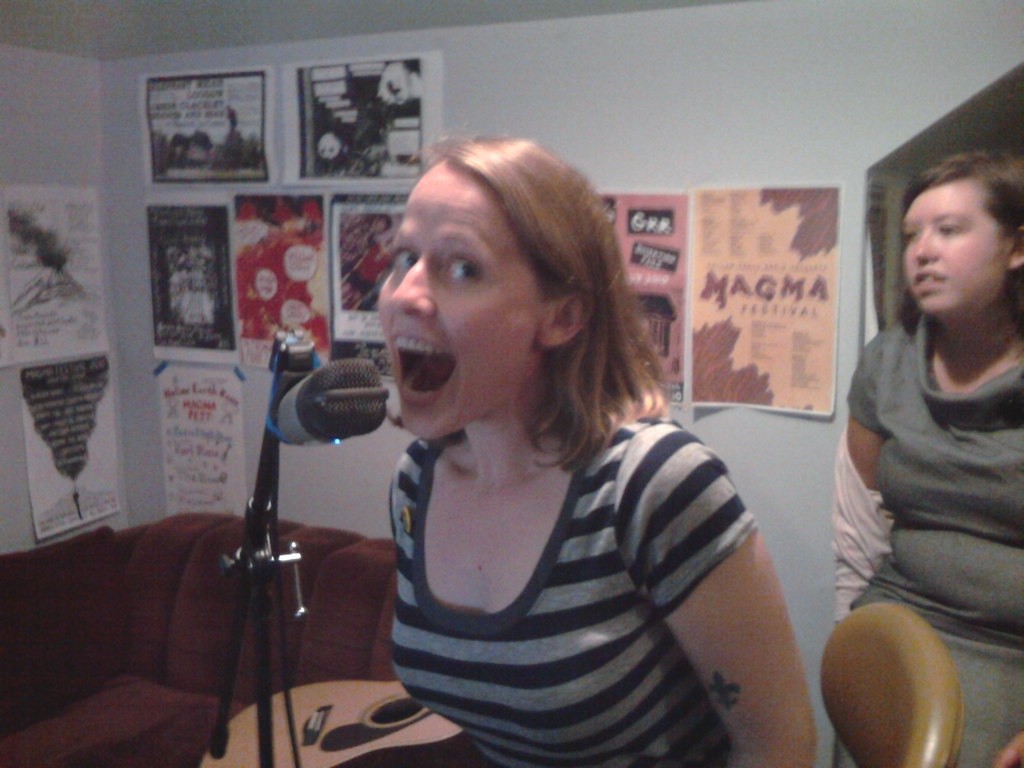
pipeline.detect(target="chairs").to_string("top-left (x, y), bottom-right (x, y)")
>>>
top-left (820, 599), bottom-right (967, 768)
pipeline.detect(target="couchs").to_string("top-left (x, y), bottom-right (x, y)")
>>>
top-left (0, 514), bottom-right (396, 768)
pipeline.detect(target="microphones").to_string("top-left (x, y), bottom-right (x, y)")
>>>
top-left (268, 356), bottom-right (392, 451)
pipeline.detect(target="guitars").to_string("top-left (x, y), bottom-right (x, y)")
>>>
top-left (200, 676), bottom-right (479, 768)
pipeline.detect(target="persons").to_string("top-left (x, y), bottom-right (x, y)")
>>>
top-left (834, 145), bottom-right (1024, 768)
top-left (377, 141), bottom-right (814, 768)
